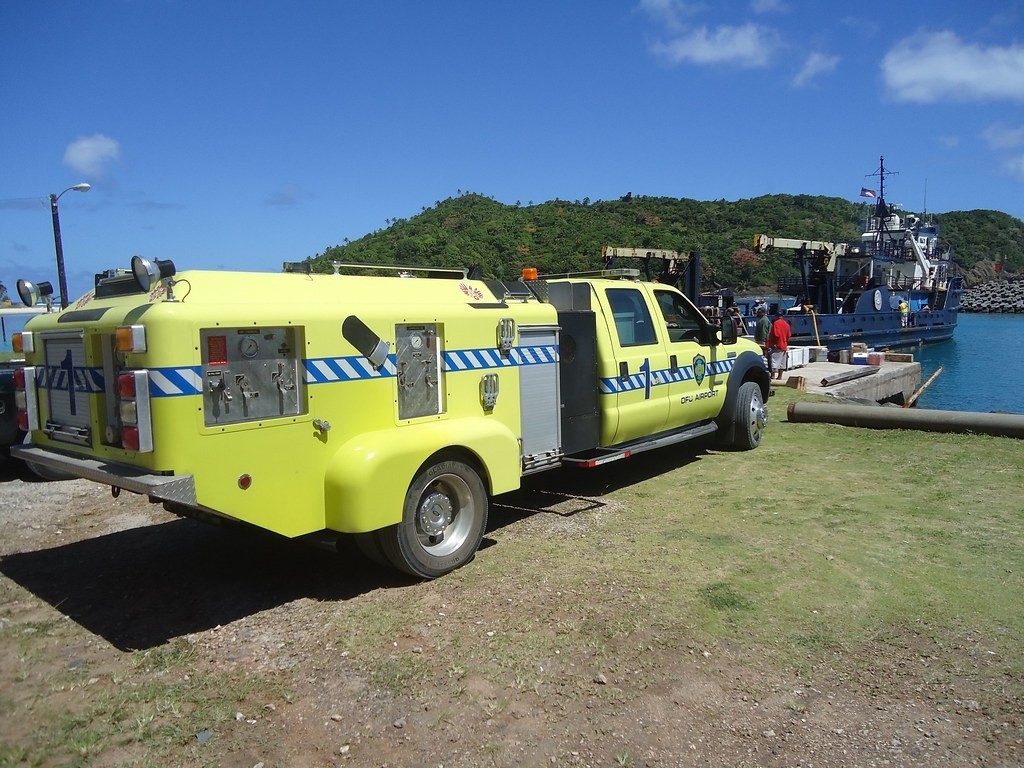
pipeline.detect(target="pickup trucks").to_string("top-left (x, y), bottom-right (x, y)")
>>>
top-left (7, 259), bottom-right (776, 582)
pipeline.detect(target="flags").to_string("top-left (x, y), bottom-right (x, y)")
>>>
top-left (859, 188), bottom-right (876, 198)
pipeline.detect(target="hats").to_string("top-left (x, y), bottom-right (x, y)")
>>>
top-left (755, 300), bottom-right (759, 303)
top-left (758, 307), bottom-right (765, 313)
top-left (774, 310), bottom-right (783, 316)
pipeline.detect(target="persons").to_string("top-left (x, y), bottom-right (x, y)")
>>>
top-left (767, 310), bottom-right (791, 380)
top-left (754, 307), bottom-right (772, 356)
top-left (897, 300), bottom-right (909, 328)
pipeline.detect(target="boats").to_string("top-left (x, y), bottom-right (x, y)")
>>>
top-left (650, 154), bottom-right (964, 355)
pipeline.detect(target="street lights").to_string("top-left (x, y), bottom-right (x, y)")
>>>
top-left (50, 183), bottom-right (92, 311)
